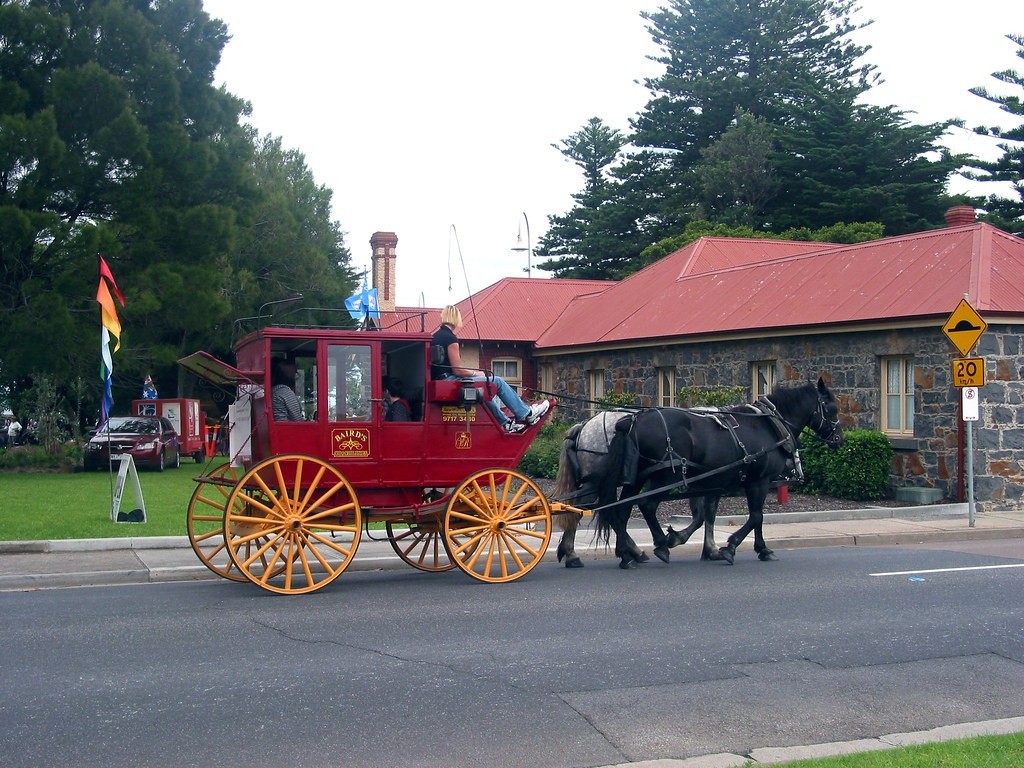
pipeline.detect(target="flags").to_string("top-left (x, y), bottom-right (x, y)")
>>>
top-left (344, 274), bottom-right (381, 320)
top-left (89, 324), bottom-right (114, 434)
top-left (99, 256), bottom-right (125, 308)
top-left (140, 373), bottom-right (158, 415)
top-left (95, 276), bottom-right (122, 354)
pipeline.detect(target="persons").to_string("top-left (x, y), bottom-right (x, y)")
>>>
top-left (270, 356), bottom-right (305, 422)
top-left (4, 416), bottom-right (38, 449)
top-left (429, 304), bottom-right (551, 435)
top-left (381, 375), bottom-right (413, 422)
top-left (346, 407), bottom-right (357, 418)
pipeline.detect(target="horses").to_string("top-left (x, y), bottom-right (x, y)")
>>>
top-left (582, 377), bottom-right (846, 568)
top-left (547, 404), bottom-right (804, 568)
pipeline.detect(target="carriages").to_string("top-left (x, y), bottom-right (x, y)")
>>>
top-left (185, 297), bottom-right (852, 593)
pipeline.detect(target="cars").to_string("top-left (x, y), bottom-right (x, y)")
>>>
top-left (0, 425), bottom-right (40, 448)
top-left (82, 414), bottom-right (182, 472)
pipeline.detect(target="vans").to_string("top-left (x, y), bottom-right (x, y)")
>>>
top-left (132, 395), bottom-right (208, 465)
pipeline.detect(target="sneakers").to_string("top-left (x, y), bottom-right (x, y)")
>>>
top-left (503, 420), bottom-right (526, 433)
top-left (526, 399), bottom-right (550, 426)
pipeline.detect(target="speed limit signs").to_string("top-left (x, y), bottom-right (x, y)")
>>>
top-left (953, 356), bottom-right (985, 387)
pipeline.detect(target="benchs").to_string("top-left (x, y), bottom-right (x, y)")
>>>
top-left (431, 379), bottom-right (496, 402)
top-left (401, 386), bottom-right (425, 421)
